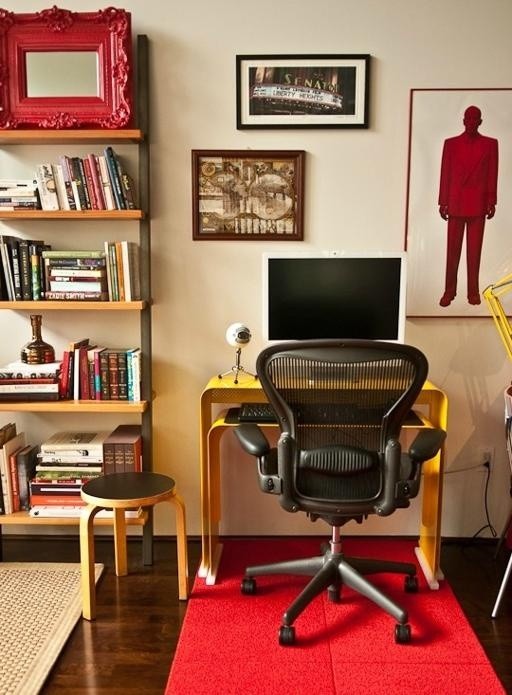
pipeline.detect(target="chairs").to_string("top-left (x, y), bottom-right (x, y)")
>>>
top-left (235, 336), bottom-right (444, 643)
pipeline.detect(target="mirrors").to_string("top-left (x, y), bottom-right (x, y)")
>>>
top-left (0, 7), bottom-right (133, 130)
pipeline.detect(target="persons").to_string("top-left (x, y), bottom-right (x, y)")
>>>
top-left (438, 106), bottom-right (498, 309)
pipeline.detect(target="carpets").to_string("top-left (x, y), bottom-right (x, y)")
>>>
top-left (3, 565), bottom-right (107, 695)
top-left (168, 537), bottom-right (506, 694)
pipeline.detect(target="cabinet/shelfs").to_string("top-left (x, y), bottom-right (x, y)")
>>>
top-left (2, 128), bottom-right (165, 564)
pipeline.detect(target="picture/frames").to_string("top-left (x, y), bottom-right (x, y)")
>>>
top-left (192, 53), bottom-right (370, 243)
top-left (404, 85), bottom-right (510, 317)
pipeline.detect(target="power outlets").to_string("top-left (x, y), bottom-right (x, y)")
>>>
top-left (475, 447), bottom-right (494, 475)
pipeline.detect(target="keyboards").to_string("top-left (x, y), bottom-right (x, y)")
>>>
top-left (238, 402), bottom-right (359, 423)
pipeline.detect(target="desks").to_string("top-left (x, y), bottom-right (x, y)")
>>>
top-left (201, 374), bottom-right (447, 591)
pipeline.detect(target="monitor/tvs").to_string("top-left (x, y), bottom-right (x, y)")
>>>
top-left (262, 250), bottom-right (408, 382)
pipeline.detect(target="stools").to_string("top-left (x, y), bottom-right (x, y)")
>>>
top-left (73, 468), bottom-right (189, 622)
